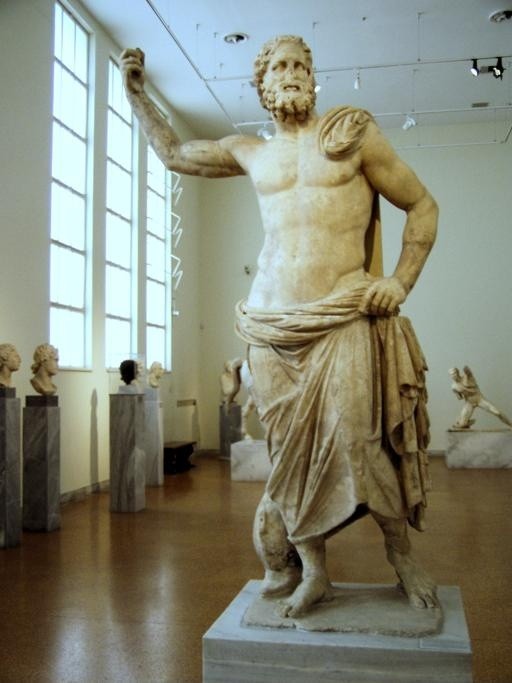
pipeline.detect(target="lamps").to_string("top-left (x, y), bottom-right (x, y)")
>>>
top-left (351, 69), bottom-right (364, 95)
top-left (255, 127), bottom-right (275, 145)
top-left (398, 112), bottom-right (421, 133)
top-left (465, 55), bottom-right (508, 78)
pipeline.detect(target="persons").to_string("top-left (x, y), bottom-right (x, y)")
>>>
top-left (0, 342), bottom-right (21, 387)
top-left (119, 359), bottom-right (139, 385)
top-left (148, 360), bottom-right (163, 387)
top-left (449, 364), bottom-right (512, 429)
top-left (119, 33), bottom-right (437, 615)
top-left (29, 342), bottom-right (59, 394)
